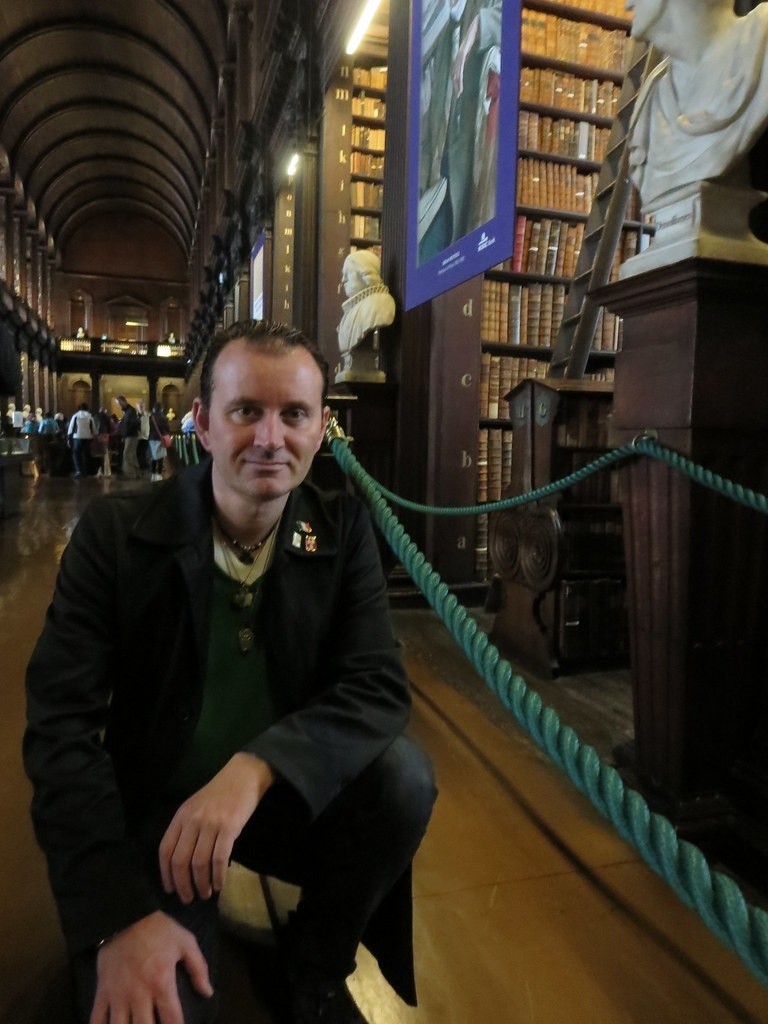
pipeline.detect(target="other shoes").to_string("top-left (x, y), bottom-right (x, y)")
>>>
top-left (75, 472), bottom-right (90, 480)
top-left (116, 473), bottom-right (141, 480)
top-left (97, 467), bottom-right (103, 479)
top-left (268, 940), bottom-right (361, 1024)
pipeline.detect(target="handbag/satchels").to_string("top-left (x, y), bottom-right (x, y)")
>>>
top-left (161, 434), bottom-right (172, 448)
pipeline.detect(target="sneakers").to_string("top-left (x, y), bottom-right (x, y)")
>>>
top-left (151, 474), bottom-right (163, 482)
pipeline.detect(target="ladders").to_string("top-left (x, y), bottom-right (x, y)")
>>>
top-left (547, 37), bottom-right (666, 379)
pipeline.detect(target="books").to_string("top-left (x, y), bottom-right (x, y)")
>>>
top-left (471, 0), bottom-right (656, 664)
top-left (349, 60), bottom-right (389, 285)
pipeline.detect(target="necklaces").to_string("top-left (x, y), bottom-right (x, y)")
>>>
top-left (209, 501), bottom-right (282, 655)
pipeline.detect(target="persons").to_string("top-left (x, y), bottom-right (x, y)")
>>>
top-left (332, 249), bottom-right (394, 384)
top-left (21, 318), bottom-right (440, 1024)
top-left (19, 393), bottom-right (199, 484)
top-left (6, 402), bottom-right (44, 424)
top-left (418, 1), bottom-right (501, 271)
top-left (612, 0), bottom-right (768, 283)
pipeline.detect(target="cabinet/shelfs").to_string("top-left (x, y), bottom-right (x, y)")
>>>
top-left (206, 0), bottom-right (768, 894)
